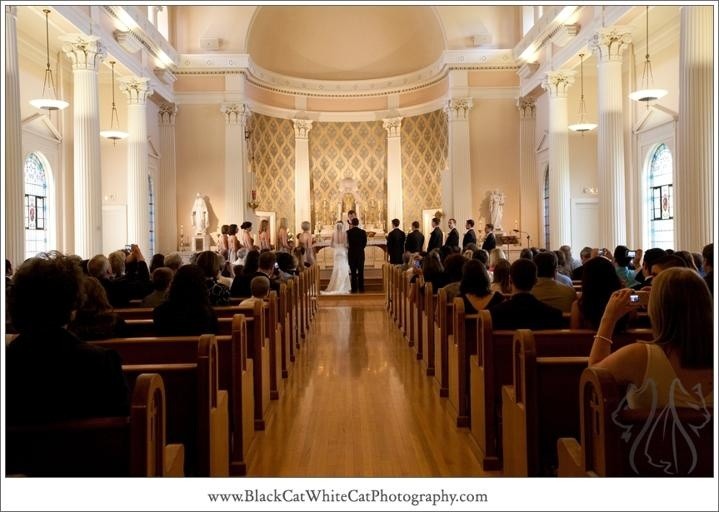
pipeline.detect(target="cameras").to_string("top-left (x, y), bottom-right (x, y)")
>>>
top-left (598, 249), bottom-right (604, 255)
top-left (630, 294), bottom-right (640, 302)
top-left (625, 250), bottom-right (636, 258)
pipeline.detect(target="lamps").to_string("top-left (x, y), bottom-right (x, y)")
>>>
top-left (628, 7), bottom-right (669, 112)
top-left (30, 9), bottom-right (71, 119)
top-left (100, 60), bottom-right (128, 146)
top-left (568, 53), bottom-right (598, 137)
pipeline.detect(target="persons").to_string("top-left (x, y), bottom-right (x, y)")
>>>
top-left (6, 213), bottom-right (326, 479)
top-left (346, 211), bottom-right (358, 225)
top-left (326, 219), bottom-right (352, 293)
top-left (585, 264), bottom-right (713, 410)
top-left (346, 217), bottom-right (369, 293)
top-left (5, 251), bottom-right (130, 477)
top-left (381, 216), bottom-right (712, 477)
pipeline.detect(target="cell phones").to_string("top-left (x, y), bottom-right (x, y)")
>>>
top-left (274, 262), bottom-right (279, 269)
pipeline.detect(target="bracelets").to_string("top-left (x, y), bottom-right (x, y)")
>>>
top-left (594, 333), bottom-right (615, 346)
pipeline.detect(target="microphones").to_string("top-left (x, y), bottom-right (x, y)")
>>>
top-left (513, 229), bottom-right (519, 232)
